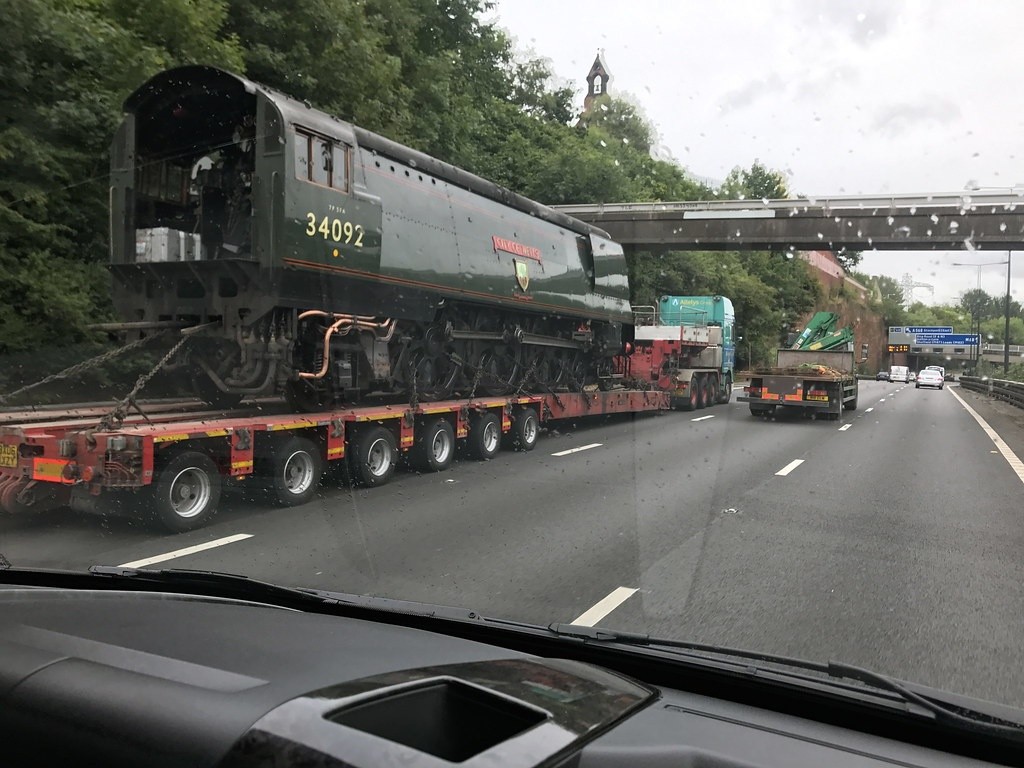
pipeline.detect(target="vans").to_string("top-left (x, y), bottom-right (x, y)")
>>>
top-left (925, 366), bottom-right (944, 379)
top-left (890, 366), bottom-right (910, 384)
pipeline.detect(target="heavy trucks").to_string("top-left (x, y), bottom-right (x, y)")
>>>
top-left (0, 292), bottom-right (744, 537)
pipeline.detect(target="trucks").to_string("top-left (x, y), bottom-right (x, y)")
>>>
top-left (736, 331), bottom-right (868, 420)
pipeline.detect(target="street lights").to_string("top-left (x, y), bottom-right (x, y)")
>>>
top-left (953, 261), bottom-right (1009, 368)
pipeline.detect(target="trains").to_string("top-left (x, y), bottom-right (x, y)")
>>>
top-left (102, 60), bottom-right (636, 413)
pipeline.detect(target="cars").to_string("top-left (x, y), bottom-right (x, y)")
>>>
top-left (876, 372), bottom-right (889, 381)
top-left (909, 372), bottom-right (917, 382)
top-left (944, 373), bottom-right (955, 382)
top-left (915, 369), bottom-right (944, 390)
